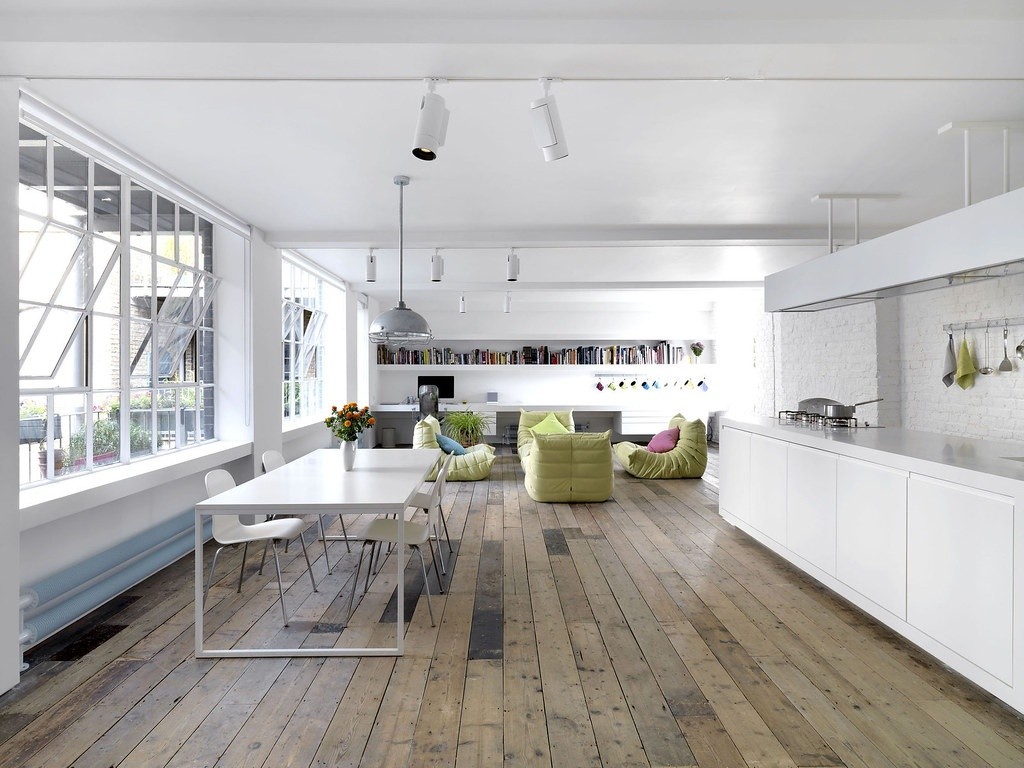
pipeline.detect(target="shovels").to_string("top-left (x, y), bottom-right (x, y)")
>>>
top-left (999, 330), bottom-right (1012, 371)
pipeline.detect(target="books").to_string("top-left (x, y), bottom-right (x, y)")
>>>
top-left (377, 342), bottom-right (684, 365)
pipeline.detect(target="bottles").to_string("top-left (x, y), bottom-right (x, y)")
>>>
top-left (416, 398), bottom-right (419, 404)
top-left (411, 396), bottom-right (413, 404)
top-left (407, 396), bottom-right (410, 404)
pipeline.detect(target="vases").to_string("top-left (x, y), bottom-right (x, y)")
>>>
top-left (340, 440), bottom-right (357, 471)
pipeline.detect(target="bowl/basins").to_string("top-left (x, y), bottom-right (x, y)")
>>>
top-left (462, 402), bottom-right (467, 404)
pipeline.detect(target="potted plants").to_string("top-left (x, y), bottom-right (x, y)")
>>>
top-left (442, 405), bottom-right (495, 448)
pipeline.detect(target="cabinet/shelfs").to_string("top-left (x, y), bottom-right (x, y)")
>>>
top-left (719, 416), bottom-right (1023, 715)
top-left (371, 364), bottom-right (714, 412)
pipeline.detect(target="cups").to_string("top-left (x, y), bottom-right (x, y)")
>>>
top-left (400, 401), bottom-right (405, 405)
top-left (607, 382), bottom-right (616, 391)
top-left (619, 382), bottom-right (627, 389)
top-left (630, 381), bottom-right (639, 389)
top-left (596, 382), bottom-right (604, 391)
top-left (697, 381), bottom-right (708, 392)
top-left (652, 381), bottom-right (660, 389)
top-left (674, 382), bottom-right (682, 389)
top-left (664, 383), bottom-right (673, 390)
top-left (684, 380), bottom-right (694, 389)
top-left (641, 382), bottom-right (650, 390)
top-left (688, 357), bottom-right (693, 364)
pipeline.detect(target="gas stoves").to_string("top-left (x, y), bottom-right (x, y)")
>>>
top-left (774, 410), bottom-right (886, 429)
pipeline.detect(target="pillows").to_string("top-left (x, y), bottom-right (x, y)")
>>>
top-left (435, 433), bottom-right (465, 456)
top-left (647, 426), bottom-right (680, 452)
top-left (528, 412), bottom-right (569, 437)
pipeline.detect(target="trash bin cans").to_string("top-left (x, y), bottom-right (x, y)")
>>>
top-left (382, 428), bottom-right (395, 448)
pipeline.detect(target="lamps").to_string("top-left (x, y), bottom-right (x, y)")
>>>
top-left (368, 175), bottom-right (434, 344)
top-left (460, 296), bottom-right (465, 313)
top-left (507, 248), bottom-right (520, 281)
top-left (504, 291), bottom-right (511, 313)
top-left (531, 77), bottom-right (568, 162)
top-left (431, 248), bottom-right (444, 282)
top-left (411, 78), bottom-right (451, 161)
top-left (367, 248), bottom-right (376, 282)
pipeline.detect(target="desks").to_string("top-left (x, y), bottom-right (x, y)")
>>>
top-left (195, 448), bottom-right (443, 658)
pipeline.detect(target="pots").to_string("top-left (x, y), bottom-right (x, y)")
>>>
top-left (824, 399), bottom-right (885, 417)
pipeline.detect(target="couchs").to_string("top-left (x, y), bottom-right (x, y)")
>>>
top-left (517, 407), bottom-right (614, 503)
top-left (413, 414), bottom-right (496, 481)
top-left (614, 413), bottom-right (708, 478)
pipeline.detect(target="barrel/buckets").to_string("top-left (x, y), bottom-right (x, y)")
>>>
top-left (382, 429), bottom-right (395, 447)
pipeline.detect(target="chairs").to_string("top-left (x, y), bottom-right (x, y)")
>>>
top-left (204, 449), bottom-right (351, 628)
top-left (343, 449), bottom-right (456, 628)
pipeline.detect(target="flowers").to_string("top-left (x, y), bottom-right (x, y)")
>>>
top-left (323, 402), bottom-right (376, 443)
top-left (691, 342), bottom-right (704, 356)
